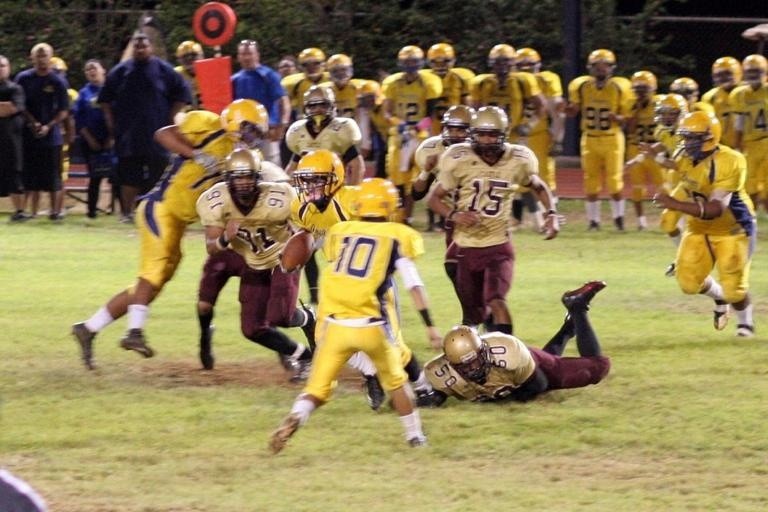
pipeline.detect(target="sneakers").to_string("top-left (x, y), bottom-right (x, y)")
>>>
top-left (289, 342), bottom-right (314, 381)
top-left (615, 226), bottom-right (627, 235)
top-left (361, 371), bottom-right (388, 412)
top-left (636, 219), bottom-right (647, 232)
top-left (506, 218), bottom-right (519, 233)
top-left (195, 323), bottom-right (216, 371)
top-left (18, 212), bottom-right (34, 222)
top-left (277, 352), bottom-right (300, 375)
top-left (296, 295), bottom-right (321, 356)
top-left (119, 327), bottom-right (155, 359)
top-left (709, 291), bottom-right (731, 330)
top-left (406, 434), bottom-right (428, 451)
top-left (86, 209), bottom-right (99, 219)
top-left (52, 212), bottom-right (68, 222)
top-left (69, 320), bottom-right (98, 373)
top-left (661, 260), bottom-right (677, 278)
top-left (735, 313), bottom-right (757, 338)
top-left (265, 413), bottom-right (303, 458)
top-left (561, 279), bottom-right (607, 316)
top-left (11, 210), bottom-right (21, 223)
top-left (417, 385), bottom-right (444, 410)
top-left (583, 219), bottom-right (601, 235)
top-left (29, 212), bottom-right (38, 219)
top-left (105, 205), bottom-right (114, 214)
top-left (119, 212), bottom-right (138, 225)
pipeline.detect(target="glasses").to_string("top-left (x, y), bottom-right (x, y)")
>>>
top-left (236, 38), bottom-right (257, 46)
top-left (84, 65), bottom-right (98, 73)
top-left (0, 61), bottom-right (9, 71)
top-left (131, 44), bottom-right (148, 50)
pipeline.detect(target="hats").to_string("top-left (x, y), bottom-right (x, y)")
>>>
top-left (276, 54), bottom-right (295, 70)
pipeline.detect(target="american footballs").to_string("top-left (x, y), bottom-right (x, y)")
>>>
top-left (281, 230), bottom-right (316, 274)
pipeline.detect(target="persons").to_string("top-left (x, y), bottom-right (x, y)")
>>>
top-left (265, 177), bottom-right (445, 454)
top-left (353, 80), bottom-right (390, 141)
top-left (627, 96), bottom-right (692, 275)
top-left (232, 41), bottom-right (291, 168)
top-left (415, 280), bottom-right (610, 403)
top-left (421, 44), bottom-right (471, 108)
top-left (411, 104), bottom-right (477, 281)
top-left (282, 85), bottom-right (365, 186)
top-left (71, 100), bottom-right (268, 369)
top-left (194, 149), bottom-right (317, 379)
top-left (50, 59), bottom-right (81, 218)
top-left (295, 49), bottom-right (333, 116)
top-left (627, 72), bottom-right (670, 233)
top-left (285, 147), bottom-right (446, 409)
top-left (276, 54), bottom-right (297, 78)
top-left (72, 60), bottom-right (125, 218)
top-left (467, 45), bottom-right (545, 148)
top-left (12, 43), bottom-right (72, 221)
top-left (709, 57), bottom-right (744, 151)
top-left (98, 34), bottom-right (196, 221)
top-left (0, 54), bottom-right (27, 220)
top-left (669, 79), bottom-right (712, 115)
top-left (731, 53), bottom-right (768, 210)
top-left (317, 53), bottom-right (370, 183)
top-left (557, 49), bottom-right (637, 233)
top-left (174, 41), bottom-right (208, 108)
top-left (377, 45), bottom-right (445, 220)
top-left (426, 105), bottom-right (559, 335)
top-left (639, 109), bottom-right (758, 339)
top-left (510, 46), bottom-right (567, 232)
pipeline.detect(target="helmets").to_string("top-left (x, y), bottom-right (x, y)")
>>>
top-left (48, 56), bottom-right (69, 77)
top-left (741, 55), bottom-right (765, 88)
top-left (300, 84), bottom-right (338, 122)
top-left (442, 326), bottom-right (494, 386)
top-left (218, 97), bottom-right (272, 148)
top-left (347, 176), bottom-right (402, 221)
top-left (584, 48), bottom-right (619, 90)
top-left (467, 105), bottom-right (513, 159)
top-left (295, 46), bottom-right (325, 83)
top-left (325, 53), bottom-right (354, 86)
top-left (669, 77), bottom-right (699, 106)
top-left (485, 43), bottom-right (516, 82)
top-left (673, 110), bottom-right (724, 165)
top-left (395, 45), bottom-right (425, 76)
top-left (436, 104), bottom-right (476, 145)
top-left (354, 78), bottom-right (382, 114)
top-left (172, 38), bottom-right (204, 73)
top-left (221, 146), bottom-right (267, 205)
top-left (649, 94), bottom-right (688, 132)
top-left (426, 42), bottom-right (458, 77)
top-left (291, 146), bottom-right (346, 210)
top-left (710, 57), bottom-right (741, 92)
top-left (628, 71), bottom-right (658, 103)
top-left (513, 48), bottom-right (543, 76)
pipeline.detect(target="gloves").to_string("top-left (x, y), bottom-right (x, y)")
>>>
top-left (191, 151), bottom-right (218, 174)
